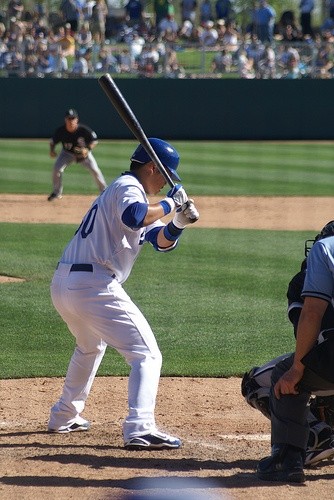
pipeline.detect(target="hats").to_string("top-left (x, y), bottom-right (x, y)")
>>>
top-left (65, 109), bottom-right (77, 118)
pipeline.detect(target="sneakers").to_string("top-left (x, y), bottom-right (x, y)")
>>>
top-left (48, 418), bottom-right (91, 433)
top-left (257, 456), bottom-right (306, 483)
top-left (305, 435), bottom-right (334, 466)
top-left (124, 431), bottom-right (181, 450)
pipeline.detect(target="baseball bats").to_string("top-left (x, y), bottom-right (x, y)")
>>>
top-left (99, 73), bottom-right (198, 223)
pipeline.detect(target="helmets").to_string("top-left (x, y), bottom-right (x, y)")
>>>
top-left (131, 138), bottom-right (182, 183)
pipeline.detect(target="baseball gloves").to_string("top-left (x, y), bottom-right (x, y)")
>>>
top-left (74, 145), bottom-right (91, 162)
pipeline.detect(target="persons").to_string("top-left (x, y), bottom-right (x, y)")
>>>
top-left (0, 0), bottom-right (334, 79)
top-left (50, 139), bottom-right (199, 449)
top-left (241, 220), bottom-right (334, 483)
top-left (48, 108), bottom-right (107, 200)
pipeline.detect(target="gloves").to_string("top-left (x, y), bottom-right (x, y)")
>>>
top-left (172, 199), bottom-right (199, 229)
top-left (162, 183), bottom-right (189, 214)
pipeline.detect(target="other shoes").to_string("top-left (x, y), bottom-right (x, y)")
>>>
top-left (48, 193), bottom-right (62, 201)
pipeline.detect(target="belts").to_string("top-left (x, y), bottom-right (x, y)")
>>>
top-left (70, 264), bottom-right (116, 278)
top-left (322, 329), bottom-right (334, 340)
